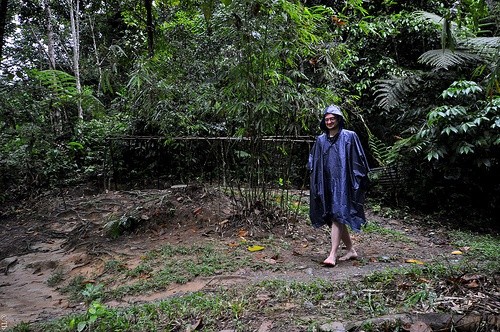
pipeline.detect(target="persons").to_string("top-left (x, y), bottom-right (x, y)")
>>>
top-left (308, 106), bottom-right (366, 265)
